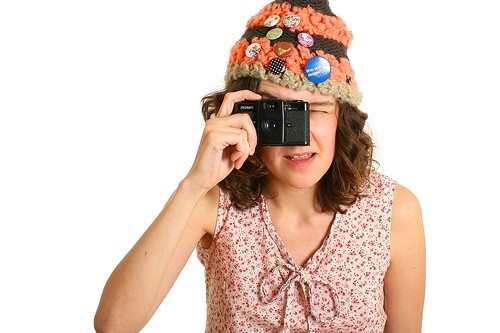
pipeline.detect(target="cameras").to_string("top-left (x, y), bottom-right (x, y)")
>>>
top-left (234, 99), bottom-right (308, 147)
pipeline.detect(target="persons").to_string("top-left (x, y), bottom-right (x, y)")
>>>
top-left (93, 0), bottom-right (425, 331)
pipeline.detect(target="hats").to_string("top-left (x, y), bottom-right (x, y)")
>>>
top-left (223, 0), bottom-right (362, 108)
